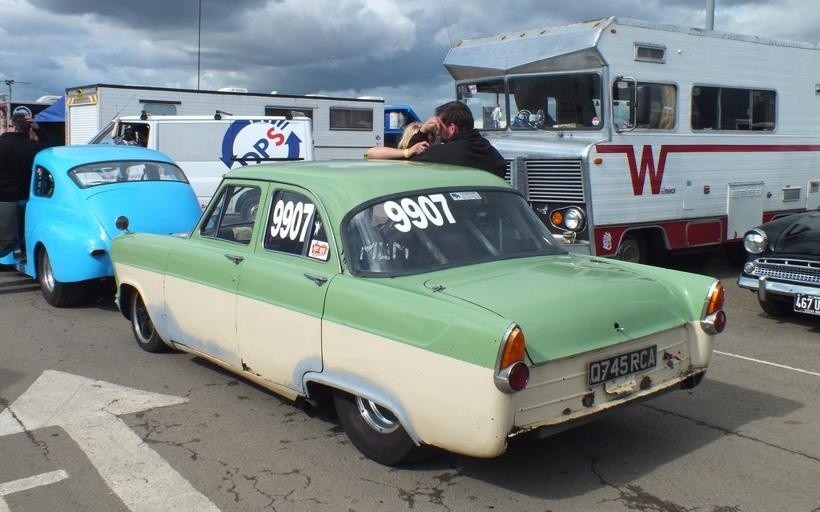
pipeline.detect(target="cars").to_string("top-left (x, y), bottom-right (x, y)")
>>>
top-left (108, 160), bottom-right (727, 469)
top-left (1, 143), bottom-right (216, 308)
top-left (737, 210), bottom-right (820, 318)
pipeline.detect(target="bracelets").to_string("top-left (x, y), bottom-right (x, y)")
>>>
top-left (404, 149), bottom-right (409, 159)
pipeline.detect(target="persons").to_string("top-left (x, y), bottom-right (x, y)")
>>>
top-left (111, 119), bottom-right (148, 148)
top-left (0, 112), bottom-right (56, 260)
top-left (365, 121), bottom-right (436, 160)
top-left (402, 100), bottom-right (508, 179)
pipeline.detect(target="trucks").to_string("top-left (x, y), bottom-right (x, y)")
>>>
top-left (88, 109), bottom-right (316, 210)
top-left (63, 82), bottom-right (424, 160)
top-left (444, 16), bottom-right (820, 263)
